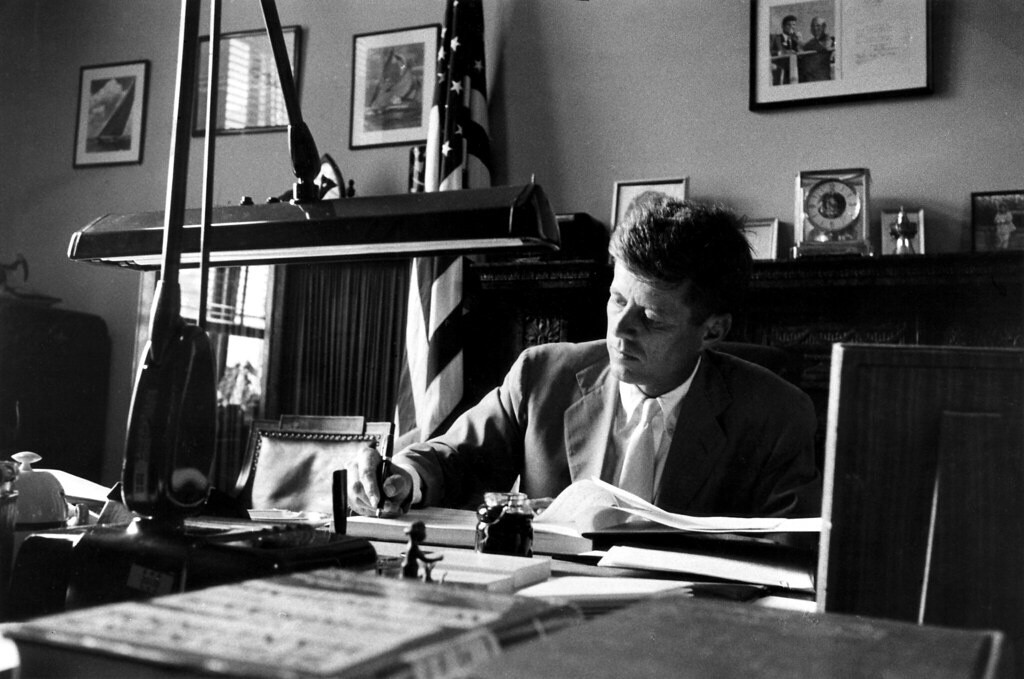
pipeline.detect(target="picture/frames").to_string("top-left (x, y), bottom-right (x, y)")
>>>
top-left (969, 191), bottom-right (1023, 257)
top-left (738, 218), bottom-right (779, 262)
top-left (350, 24), bottom-right (441, 149)
top-left (880, 210), bottom-right (925, 254)
top-left (192, 26), bottom-right (300, 136)
top-left (609, 179), bottom-right (688, 261)
top-left (749, 1), bottom-right (934, 111)
top-left (73, 59), bottom-right (149, 167)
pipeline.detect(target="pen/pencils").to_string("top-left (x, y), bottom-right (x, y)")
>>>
top-left (375, 422), bottom-right (395, 518)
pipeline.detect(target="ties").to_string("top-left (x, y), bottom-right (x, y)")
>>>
top-left (618, 396), bottom-right (662, 507)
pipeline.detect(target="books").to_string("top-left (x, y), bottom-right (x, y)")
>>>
top-left (345, 475), bottom-right (822, 559)
top-left (401, 546), bottom-right (554, 593)
top-left (0, 569), bottom-right (581, 679)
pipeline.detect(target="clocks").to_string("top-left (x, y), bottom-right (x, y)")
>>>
top-left (793, 167), bottom-right (871, 258)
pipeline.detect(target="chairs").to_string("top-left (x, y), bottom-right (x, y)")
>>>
top-left (225, 416), bottom-right (392, 515)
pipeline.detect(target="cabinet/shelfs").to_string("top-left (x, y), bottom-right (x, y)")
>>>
top-left (456, 260), bottom-right (1024, 635)
top-left (0, 301), bottom-right (111, 486)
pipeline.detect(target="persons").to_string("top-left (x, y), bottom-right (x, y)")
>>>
top-left (804, 16), bottom-right (837, 67)
top-left (769, 15), bottom-right (805, 81)
top-left (346, 189), bottom-right (824, 563)
top-left (995, 203), bottom-right (1017, 248)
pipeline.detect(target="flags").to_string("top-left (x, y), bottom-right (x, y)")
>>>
top-left (403, 0), bottom-right (496, 447)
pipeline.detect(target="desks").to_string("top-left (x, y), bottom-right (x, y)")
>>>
top-left (2, 512), bottom-right (1024, 679)
